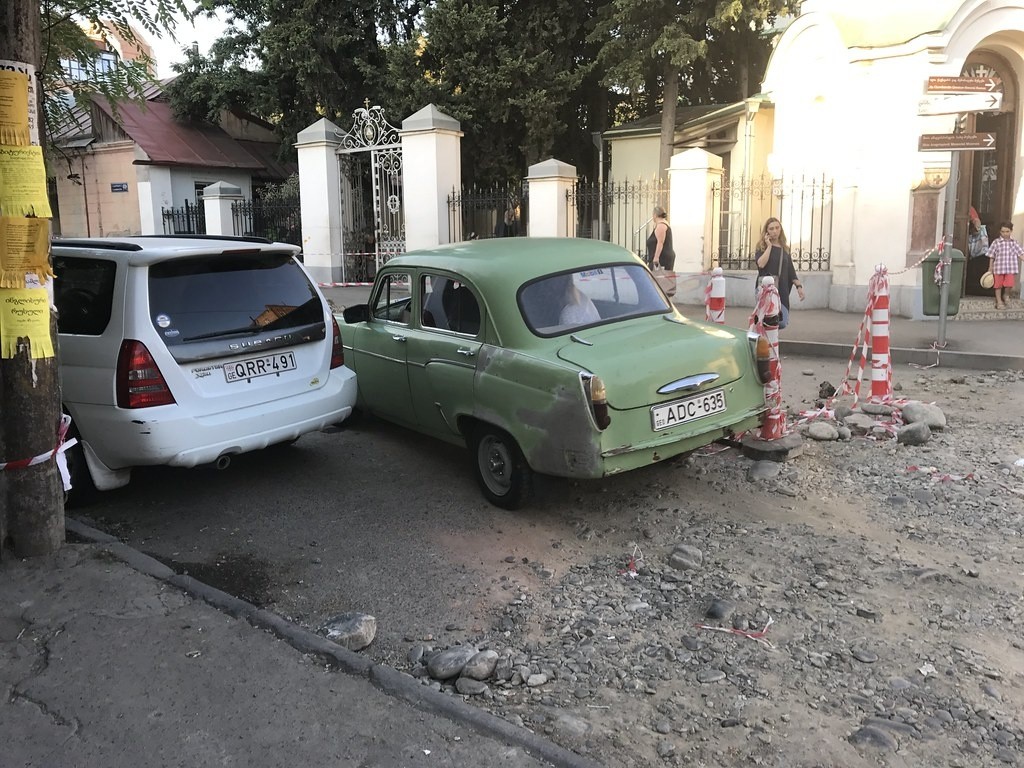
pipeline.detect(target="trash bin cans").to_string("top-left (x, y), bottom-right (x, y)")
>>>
top-left (922, 248), bottom-right (965, 315)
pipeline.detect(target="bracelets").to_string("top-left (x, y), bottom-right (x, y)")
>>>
top-left (796, 285), bottom-right (802, 289)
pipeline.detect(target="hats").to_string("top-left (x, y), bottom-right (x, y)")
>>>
top-left (980, 272), bottom-right (994, 288)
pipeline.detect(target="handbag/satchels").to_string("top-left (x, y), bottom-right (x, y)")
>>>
top-left (651, 262), bottom-right (676, 297)
top-left (967, 225), bottom-right (989, 261)
top-left (758, 276), bottom-right (779, 292)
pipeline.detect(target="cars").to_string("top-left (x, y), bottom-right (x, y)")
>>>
top-left (334, 234), bottom-right (767, 511)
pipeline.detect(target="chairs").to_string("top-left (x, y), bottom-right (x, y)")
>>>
top-left (449, 286), bottom-right (480, 334)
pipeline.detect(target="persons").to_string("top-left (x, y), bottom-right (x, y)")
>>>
top-left (754, 217), bottom-right (806, 329)
top-left (646, 207), bottom-right (676, 300)
top-left (547, 273), bottom-right (602, 326)
top-left (969, 205), bottom-right (981, 236)
top-left (494, 208), bottom-right (518, 237)
top-left (984, 221), bottom-right (1024, 310)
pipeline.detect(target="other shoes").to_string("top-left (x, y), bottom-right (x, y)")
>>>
top-left (996, 302), bottom-right (1006, 310)
top-left (1001, 293), bottom-right (1011, 304)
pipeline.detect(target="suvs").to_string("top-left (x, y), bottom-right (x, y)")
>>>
top-left (50, 232), bottom-right (360, 507)
